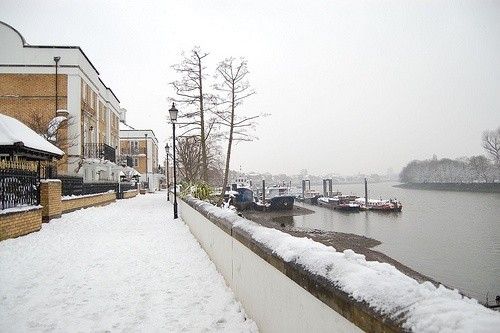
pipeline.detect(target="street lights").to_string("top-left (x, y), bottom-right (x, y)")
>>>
top-left (167, 102), bottom-right (178, 219)
top-left (165, 142), bottom-right (170, 201)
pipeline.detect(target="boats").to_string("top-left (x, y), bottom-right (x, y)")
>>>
top-left (231, 175), bottom-right (403, 213)
